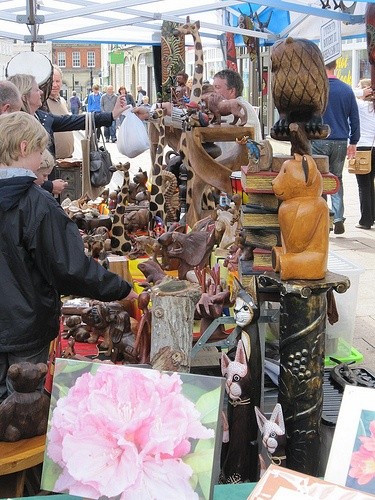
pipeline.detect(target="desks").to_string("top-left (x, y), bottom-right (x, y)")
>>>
top-left (0, 435), bottom-right (46, 498)
top-left (253, 270), bottom-right (351, 477)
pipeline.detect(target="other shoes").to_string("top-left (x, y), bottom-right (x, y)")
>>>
top-left (333, 218), bottom-right (345, 234)
top-left (355, 220), bottom-right (375, 229)
top-left (328, 209), bottom-right (335, 231)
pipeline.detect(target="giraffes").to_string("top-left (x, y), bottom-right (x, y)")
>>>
top-left (151, 103), bottom-right (167, 236)
top-left (109, 161), bottom-right (133, 256)
top-left (169, 129), bottom-right (216, 210)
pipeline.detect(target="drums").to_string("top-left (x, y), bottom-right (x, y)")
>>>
top-left (4, 50), bottom-right (53, 105)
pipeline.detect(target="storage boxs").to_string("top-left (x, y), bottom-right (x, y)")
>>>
top-left (263, 251), bottom-right (366, 366)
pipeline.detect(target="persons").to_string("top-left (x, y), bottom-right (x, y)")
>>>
top-left (0, 111), bottom-right (138, 403)
top-left (100, 85), bottom-right (119, 142)
top-left (308, 59), bottom-right (360, 232)
top-left (118, 87), bottom-right (135, 123)
top-left (88, 84), bottom-right (103, 141)
top-left (140, 95), bottom-right (152, 108)
top-left (4, 73), bottom-right (131, 193)
top-left (134, 70), bottom-right (262, 154)
top-left (174, 71), bottom-right (191, 101)
top-left (46, 64), bottom-right (73, 158)
top-left (0, 81), bottom-right (55, 184)
top-left (70, 91), bottom-right (81, 114)
top-left (349, 79), bottom-right (375, 229)
top-left (137, 85), bottom-right (146, 107)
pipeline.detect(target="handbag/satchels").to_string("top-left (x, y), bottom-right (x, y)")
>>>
top-left (116, 104), bottom-right (152, 158)
top-left (84, 112), bottom-right (114, 187)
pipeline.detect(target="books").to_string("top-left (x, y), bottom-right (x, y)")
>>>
top-left (240, 154), bottom-right (339, 272)
